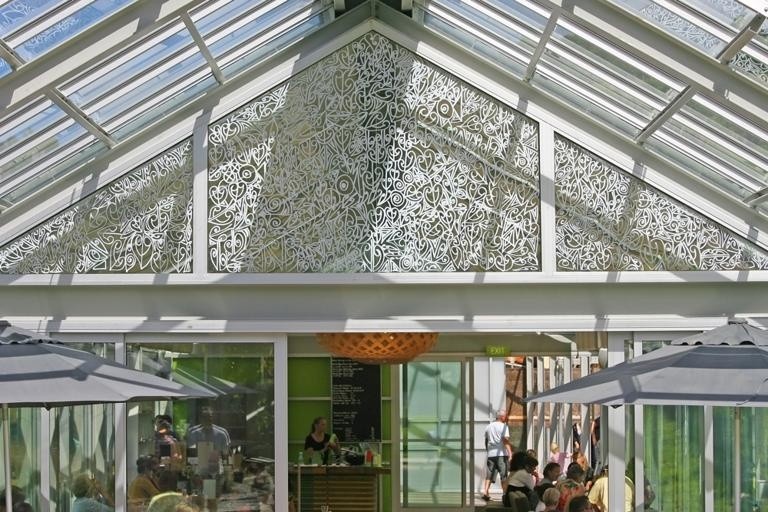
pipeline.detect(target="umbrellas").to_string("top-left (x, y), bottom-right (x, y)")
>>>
top-left (0, 319), bottom-right (261, 512)
top-left (245, 456), bottom-right (274, 463)
top-left (521, 316), bottom-right (768, 512)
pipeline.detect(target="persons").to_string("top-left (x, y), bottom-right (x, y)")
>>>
top-left (253, 463), bottom-right (273, 492)
top-left (304, 417), bottom-right (339, 465)
top-left (71, 475), bottom-right (114, 512)
top-left (481, 410), bottom-right (656, 511)
top-left (1, 485), bottom-right (25, 512)
top-left (16, 503), bottom-right (32, 512)
top-left (125, 404), bottom-right (231, 512)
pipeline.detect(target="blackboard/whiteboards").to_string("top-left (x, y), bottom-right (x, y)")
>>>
top-left (331, 357), bottom-right (383, 444)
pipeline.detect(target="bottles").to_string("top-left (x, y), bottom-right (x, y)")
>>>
top-left (297, 452), bottom-right (304, 465)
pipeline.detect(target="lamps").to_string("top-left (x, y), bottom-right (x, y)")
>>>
top-left (311, 332), bottom-right (441, 366)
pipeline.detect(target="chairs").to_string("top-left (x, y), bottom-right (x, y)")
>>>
top-left (507, 490), bottom-right (531, 511)
